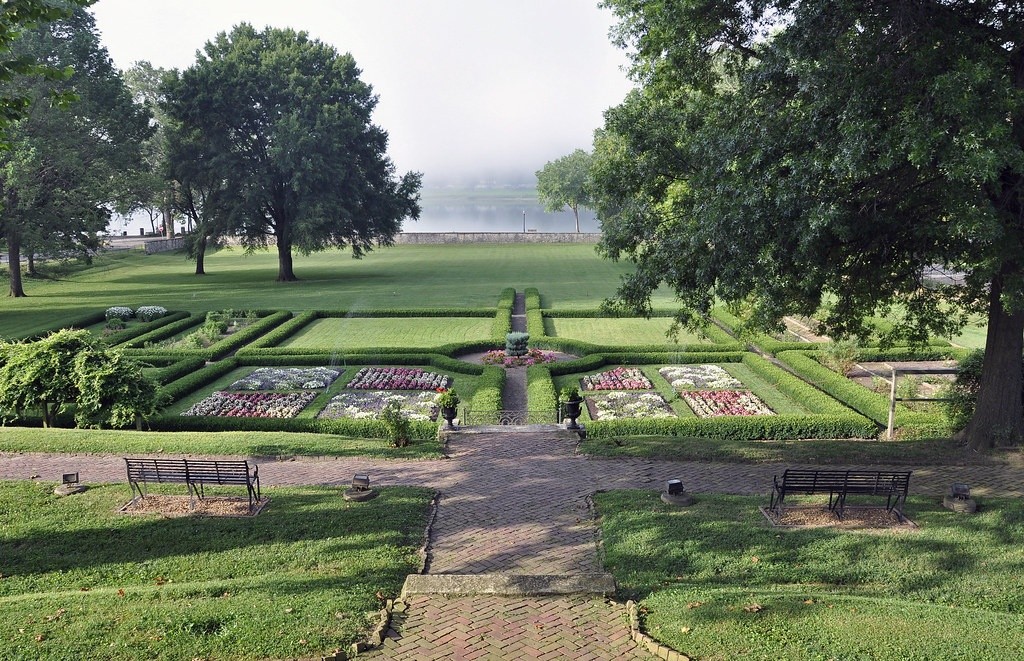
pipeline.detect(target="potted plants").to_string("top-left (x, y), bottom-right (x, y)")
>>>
top-left (438, 388), bottom-right (460, 429)
top-left (558, 388), bottom-right (585, 429)
top-left (381, 398), bottom-right (411, 447)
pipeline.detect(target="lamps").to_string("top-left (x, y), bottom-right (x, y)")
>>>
top-left (352, 474), bottom-right (372, 491)
top-left (62, 472), bottom-right (79, 488)
top-left (667, 479), bottom-right (685, 497)
top-left (951, 483), bottom-right (970, 501)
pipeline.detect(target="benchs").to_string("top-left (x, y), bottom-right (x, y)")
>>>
top-left (769, 469), bottom-right (913, 524)
top-left (123, 457), bottom-right (260, 514)
